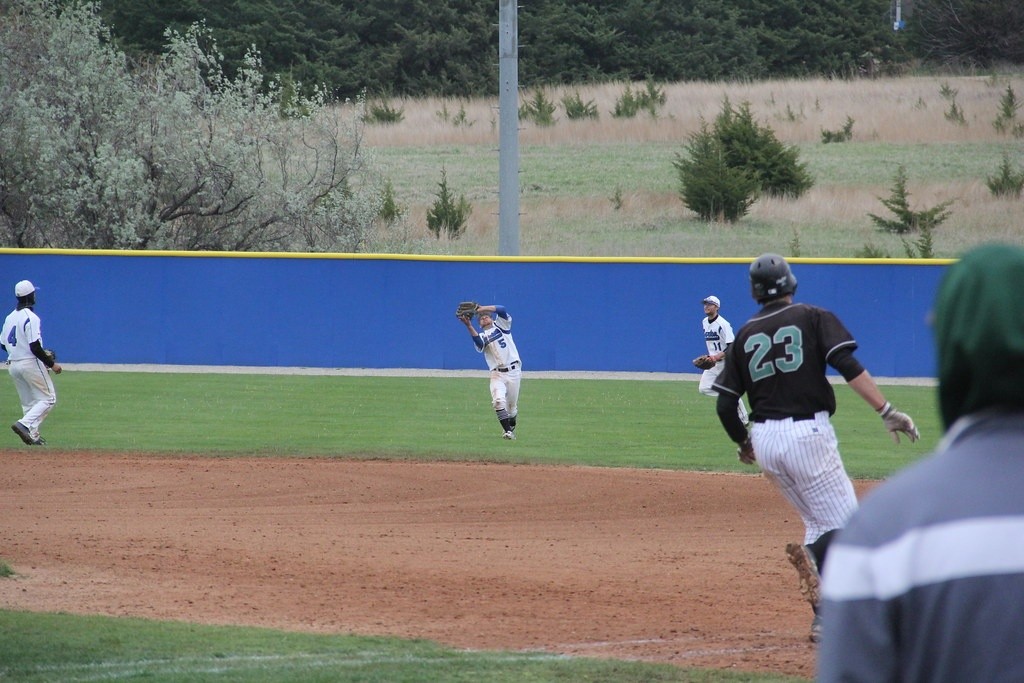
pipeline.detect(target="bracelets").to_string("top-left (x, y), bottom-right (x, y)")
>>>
top-left (877, 401), bottom-right (894, 419)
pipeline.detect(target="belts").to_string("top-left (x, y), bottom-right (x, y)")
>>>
top-left (754, 413), bottom-right (815, 424)
top-left (494, 365), bottom-right (515, 372)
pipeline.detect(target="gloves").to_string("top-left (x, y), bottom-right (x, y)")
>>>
top-left (738, 437), bottom-right (756, 464)
top-left (879, 401), bottom-right (921, 443)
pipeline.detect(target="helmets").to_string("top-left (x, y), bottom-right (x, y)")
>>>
top-left (749, 253), bottom-right (797, 305)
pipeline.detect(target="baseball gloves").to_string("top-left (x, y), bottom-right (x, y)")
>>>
top-left (692, 355), bottom-right (717, 370)
top-left (455, 301), bottom-right (480, 322)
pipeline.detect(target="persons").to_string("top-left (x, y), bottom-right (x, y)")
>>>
top-left (693, 295), bottom-right (749, 428)
top-left (710, 253), bottom-right (921, 644)
top-left (455, 302), bottom-right (522, 440)
top-left (1, 280), bottom-right (63, 445)
top-left (816, 242), bottom-right (1024, 682)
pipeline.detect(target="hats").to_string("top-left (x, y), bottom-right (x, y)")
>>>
top-left (14, 280), bottom-right (40, 298)
top-left (700, 295), bottom-right (721, 308)
top-left (477, 312), bottom-right (491, 322)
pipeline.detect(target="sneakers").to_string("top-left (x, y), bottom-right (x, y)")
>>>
top-left (34, 436), bottom-right (48, 445)
top-left (809, 617), bottom-right (827, 645)
top-left (502, 426), bottom-right (517, 440)
top-left (785, 542), bottom-right (822, 607)
top-left (11, 421), bottom-right (33, 445)
